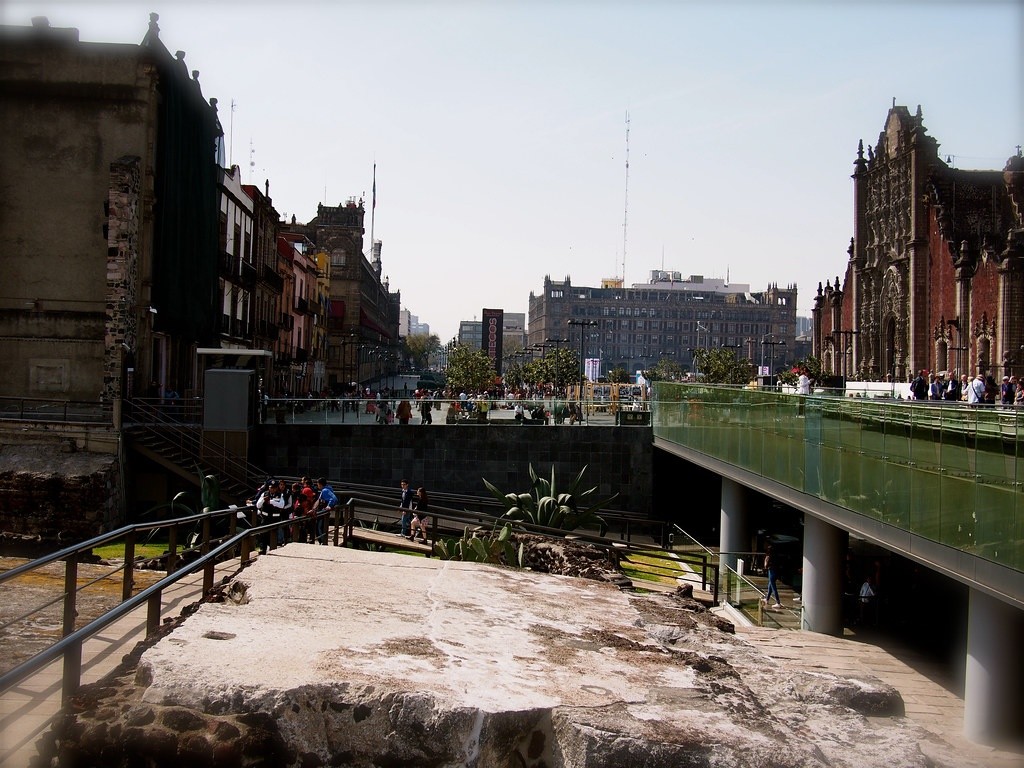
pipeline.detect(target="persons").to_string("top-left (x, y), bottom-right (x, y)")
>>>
top-left (361, 382), bottom-right (443, 425)
top-left (910, 369), bottom-right (1024, 411)
top-left (858, 576), bottom-right (876, 605)
top-left (794, 368), bottom-right (814, 418)
top-left (144, 380), bottom-right (179, 423)
top-left (847, 369), bottom-right (913, 383)
top-left (595, 393), bottom-right (641, 413)
top-left (263, 390), bottom-right (360, 424)
top-left (849, 392), bottom-right (910, 401)
top-left (760, 545), bottom-right (782, 608)
top-left (508, 382), bottom-right (584, 425)
top-left (398, 479), bottom-right (429, 545)
top-left (246, 476), bottom-right (338, 555)
top-left (448, 382), bottom-right (505, 420)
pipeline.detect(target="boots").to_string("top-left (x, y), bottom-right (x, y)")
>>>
top-left (419, 538), bottom-right (428, 545)
top-left (406, 534), bottom-right (415, 542)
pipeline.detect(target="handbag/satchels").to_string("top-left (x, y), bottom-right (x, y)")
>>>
top-left (312, 499), bottom-right (326, 512)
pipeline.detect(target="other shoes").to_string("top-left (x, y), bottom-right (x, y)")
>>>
top-left (396, 534), bottom-right (405, 537)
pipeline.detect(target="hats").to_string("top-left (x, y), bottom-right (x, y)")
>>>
top-left (268, 479), bottom-right (278, 488)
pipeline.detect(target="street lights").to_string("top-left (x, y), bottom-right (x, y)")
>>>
top-left (339, 333), bottom-right (394, 423)
top-left (721, 344), bottom-right (742, 385)
top-left (830, 329), bottom-right (859, 395)
top-left (532, 344), bottom-right (554, 399)
top-left (523, 346), bottom-right (541, 364)
top-left (686, 347), bottom-right (699, 382)
top-left (886, 348), bottom-right (902, 382)
top-left (566, 319), bottom-right (598, 425)
top-left (761, 341), bottom-right (786, 391)
top-left (514, 350), bottom-right (531, 366)
top-left (508, 354), bottom-right (522, 366)
top-left (620, 356), bottom-right (635, 375)
top-left (948, 347), bottom-right (968, 381)
top-left (660, 351), bottom-right (675, 383)
top-left (638, 354), bottom-right (652, 372)
top-left (545, 338), bottom-right (571, 399)
